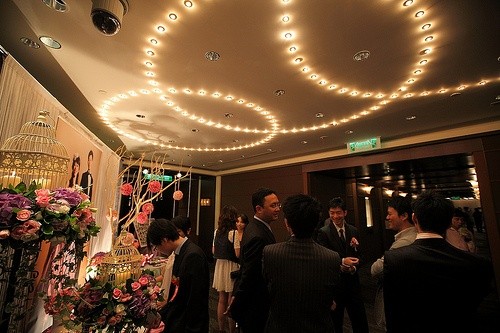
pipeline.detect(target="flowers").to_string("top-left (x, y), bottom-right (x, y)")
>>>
top-left (0, 183), bottom-right (166, 333)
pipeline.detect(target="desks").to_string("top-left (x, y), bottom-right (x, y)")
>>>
top-left (86, 255), bottom-right (167, 299)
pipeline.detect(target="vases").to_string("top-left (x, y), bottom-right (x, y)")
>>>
top-left (51, 308), bottom-right (77, 333)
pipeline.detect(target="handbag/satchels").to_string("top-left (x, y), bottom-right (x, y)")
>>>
top-left (223, 230), bottom-right (236, 261)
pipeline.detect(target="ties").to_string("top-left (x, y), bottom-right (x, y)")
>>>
top-left (339, 229), bottom-right (345, 241)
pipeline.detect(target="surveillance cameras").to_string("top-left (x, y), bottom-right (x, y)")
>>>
top-left (90, 0), bottom-right (129, 36)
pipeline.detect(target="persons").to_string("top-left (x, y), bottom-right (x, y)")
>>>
top-left (150, 216), bottom-right (192, 309)
top-left (261, 195), bottom-right (342, 333)
top-left (211, 204), bottom-right (250, 333)
top-left (317, 197), bottom-right (369, 333)
top-left (370, 195), bottom-right (419, 276)
top-left (446, 208), bottom-right (475, 252)
top-left (383, 188), bottom-right (476, 333)
top-left (226, 188), bottom-right (282, 333)
top-left (457, 206), bottom-right (483, 235)
top-left (146, 218), bottom-right (211, 333)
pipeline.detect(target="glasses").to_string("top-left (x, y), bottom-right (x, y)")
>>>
top-left (153, 244), bottom-right (159, 253)
top-left (262, 202), bottom-right (282, 209)
